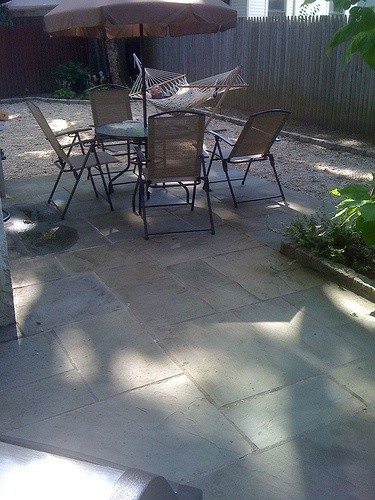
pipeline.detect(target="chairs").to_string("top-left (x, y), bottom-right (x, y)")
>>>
top-left (129, 109), bottom-right (215, 240)
top-left (88, 83), bottom-right (145, 179)
top-left (202, 108), bottom-right (293, 208)
top-left (25, 100), bottom-right (119, 220)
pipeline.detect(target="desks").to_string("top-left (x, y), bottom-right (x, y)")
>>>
top-left (94, 119), bottom-right (200, 205)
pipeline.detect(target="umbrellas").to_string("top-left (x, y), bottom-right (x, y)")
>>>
top-left (43, 0), bottom-right (239, 125)
